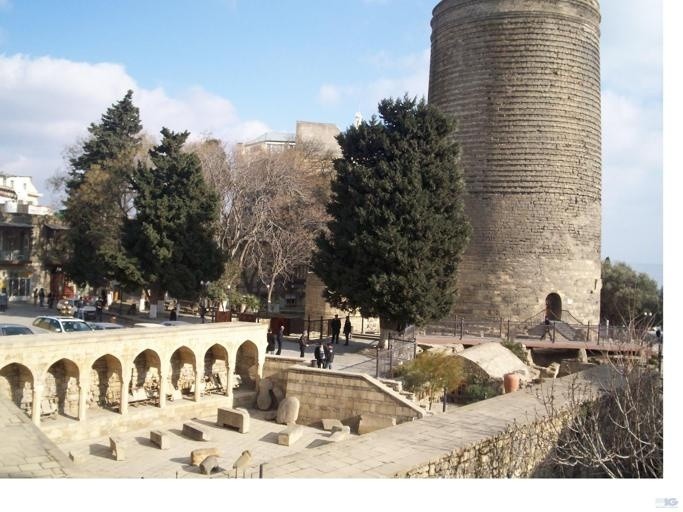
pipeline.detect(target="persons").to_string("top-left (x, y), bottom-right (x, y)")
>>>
top-left (323, 345), bottom-right (334, 370)
top-left (539, 315), bottom-right (552, 343)
top-left (298, 330), bottom-right (308, 357)
top-left (314, 342), bottom-right (326, 368)
top-left (342, 315), bottom-right (350, 346)
top-left (266, 329), bottom-right (275, 355)
top-left (331, 314), bottom-right (340, 344)
top-left (275, 325), bottom-right (285, 355)
top-left (31, 286), bottom-right (205, 326)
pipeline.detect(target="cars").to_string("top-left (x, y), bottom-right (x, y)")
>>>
top-left (1, 314), bottom-right (128, 337)
top-left (161, 320), bottom-right (194, 327)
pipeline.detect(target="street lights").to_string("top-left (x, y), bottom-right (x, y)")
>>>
top-left (372, 345), bottom-right (382, 378)
top-left (200, 279), bottom-right (210, 323)
top-left (644, 307), bottom-right (653, 349)
top-left (226, 284), bottom-right (232, 321)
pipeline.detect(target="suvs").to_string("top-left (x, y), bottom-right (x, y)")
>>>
top-left (56, 297), bottom-right (98, 319)
